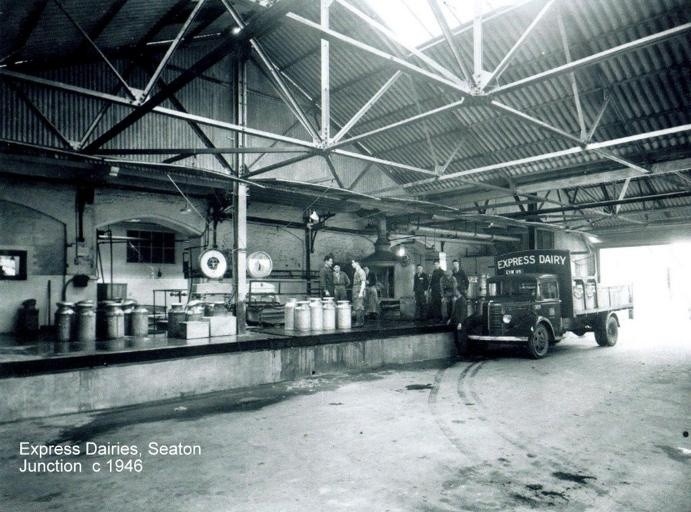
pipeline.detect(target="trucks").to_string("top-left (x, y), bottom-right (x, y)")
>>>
top-left (457, 249), bottom-right (635, 363)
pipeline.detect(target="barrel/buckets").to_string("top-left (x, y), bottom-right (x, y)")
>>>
top-left (167, 305), bottom-right (187, 338)
top-left (284, 298), bottom-right (352, 331)
top-left (132, 307), bottom-right (150, 336)
top-left (54, 305), bottom-right (77, 342)
top-left (105, 306), bottom-right (125, 339)
top-left (77, 305), bottom-right (97, 342)
top-left (208, 303), bottom-right (227, 314)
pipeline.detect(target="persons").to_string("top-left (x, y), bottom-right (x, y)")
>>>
top-left (429, 259), bottom-right (444, 322)
top-left (440, 267), bottom-right (457, 322)
top-left (445, 285), bottom-right (468, 359)
top-left (319, 255), bottom-right (334, 296)
top-left (363, 264), bottom-right (381, 320)
top-left (412, 264), bottom-right (428, 322)
top-left (350, 256), bottom-right (367, 328)
top-left (451, 259), bottom-right (470, 298)
top-left (333, 263), bottom-right (350, 300)
